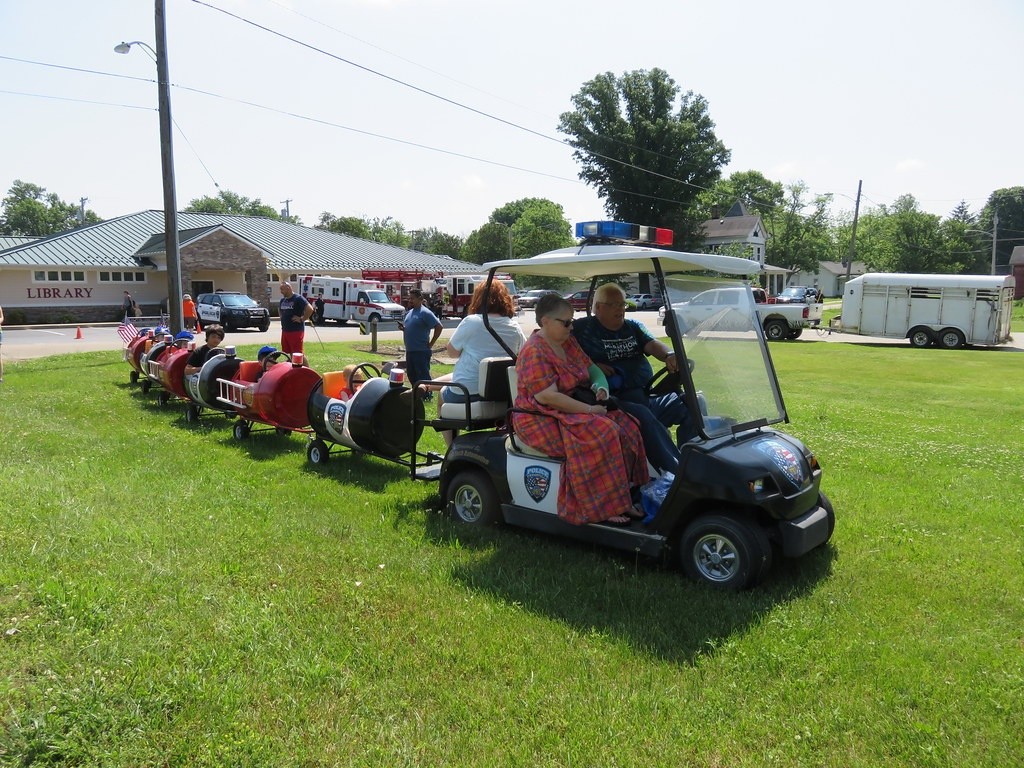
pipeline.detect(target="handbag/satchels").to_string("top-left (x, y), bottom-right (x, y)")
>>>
top-left (196, 320), bottom-right (201, 333)
top-left (571, 386), bottom-right (619, 412)
top-left (639, 470), bottom-right (676, 522)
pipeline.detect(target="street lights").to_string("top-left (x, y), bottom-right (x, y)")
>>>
top-left (114, 41), bottom-right (184, 335)
top-left (964, 212), bottom-right (999, 275)
top-left (495, 222), bottom-right (513, 278)
top-left (824, 180), bottom-right (863, 279)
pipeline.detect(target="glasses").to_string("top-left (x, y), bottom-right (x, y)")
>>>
top-left (599, 301), bottom-right (629, 309)
top-left (556, 317), bottom-right (577, 328)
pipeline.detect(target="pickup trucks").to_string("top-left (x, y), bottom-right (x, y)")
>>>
top-left (657, 288), bottom-right (824, 340)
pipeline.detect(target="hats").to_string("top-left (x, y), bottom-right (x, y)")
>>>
top-left (154, 325), bottom-right (170, 337)
top-left (258, 346), bottom-right (280, 360)
top-left (176, 330), bottom-right (194, 340)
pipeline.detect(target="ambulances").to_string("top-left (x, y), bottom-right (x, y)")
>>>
top-left (298, 275), bottom-right (407, 326)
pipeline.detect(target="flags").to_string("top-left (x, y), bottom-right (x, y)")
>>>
top-left (117, 316), bottom-right (139, 344)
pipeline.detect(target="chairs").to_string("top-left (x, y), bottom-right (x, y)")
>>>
top-left (155, 350), bottom-right (166, 364)
top-left (321, 370), bottom-right (348, 400)
top-left (144, 339), bottom-right (152, 354)
top-left (239, 360), bottom-right (263, 384)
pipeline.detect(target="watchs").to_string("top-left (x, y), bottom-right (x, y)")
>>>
top-left (666, 351), bottom-right (675, 358)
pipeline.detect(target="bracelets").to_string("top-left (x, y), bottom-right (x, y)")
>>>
top-left (589, 405), bottom-right (592, 414)
top-left (300, 317), bottom-right (303, 322)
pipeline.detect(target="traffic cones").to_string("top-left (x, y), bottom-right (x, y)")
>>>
top-left (196, 321), bottom-right (202, 333)
top-left (74, 326), bottom-right (84, 339)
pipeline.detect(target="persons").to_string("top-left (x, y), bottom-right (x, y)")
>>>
top-left (815, 290), bottom-right (824, 303)
top-left (339, 364), bottom-right (362, 402)
top-left (151, 325), bottom-right (170, 348)
top-left (183, 294), bottom-right (197, 333)
top-left (422, 299), bottom-right (427, 307)
top-left (257, 345), bottom-right (309, 382)
top-left (513, 295), bottom-right (649, 524)
top-left (434, 293), bottom-right (445, 320)
top-left (121, 291), bottom-right (133, 322)
top-left (278, 282), bottom-right (313, 354)
top-left (184, 324), bottom-right (224, 374)
top-left (398, 289), bottom-right (443, 401)
top-left (401, 278), bottom-right (527, 449)
top-left (133, 304), bottom-right (142, 322)
top-left (155, 330), bottom-right (194, 362)
top-left (315, 293), bottom-right (325, 325)
top-left (571, 283), bottom-right (706, 477)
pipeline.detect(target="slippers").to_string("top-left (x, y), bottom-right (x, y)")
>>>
top-left (602, 514), bottom-right (632, 526)
top-left (624, 504), bottom-right (648, 519)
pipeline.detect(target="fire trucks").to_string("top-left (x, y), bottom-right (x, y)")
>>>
top-left (362, 269), bottom-right (522, 319)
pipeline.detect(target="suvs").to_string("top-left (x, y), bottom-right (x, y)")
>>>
top-left (196, 290), bottom-right (270, 332)
top-left (777, 286), bottom-right (824, 304)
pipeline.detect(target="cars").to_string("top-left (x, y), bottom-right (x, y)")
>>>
top-left (517, 290), bottom-right (664, 312)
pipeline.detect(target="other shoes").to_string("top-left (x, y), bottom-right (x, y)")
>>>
top-left (400, 383), bottom-right (427, 405)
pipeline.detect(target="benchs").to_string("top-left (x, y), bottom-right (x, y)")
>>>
top-left (507, 365), bottom-right (551, 460)
top-left (434, 356), bottom-right (519, 431)
